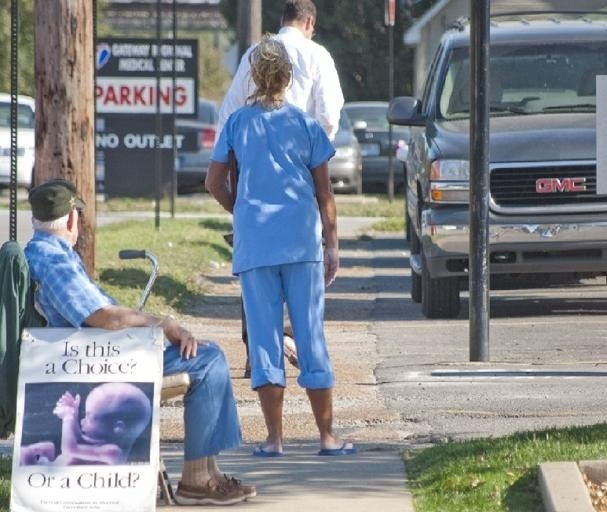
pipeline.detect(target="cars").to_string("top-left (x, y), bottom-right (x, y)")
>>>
top-left (327, 109), bottom-right (363, 194)
top-left (173, 99), bottom-right (230, 190)
top-left (343, 101), bottom-right (411, 194)
top-left (0, 93), bottom-right (35, 191)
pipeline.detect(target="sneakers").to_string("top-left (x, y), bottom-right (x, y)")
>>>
top-left (222, 474), bottom-right (256, 498)
top-left (174, 477), bottom-right (246, 505)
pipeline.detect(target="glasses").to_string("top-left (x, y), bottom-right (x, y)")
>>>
top-left (311, 22), bottom-right (319, 39)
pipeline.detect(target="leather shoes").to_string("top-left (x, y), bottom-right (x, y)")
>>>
top-left (283, 336), bottom-right (299, 369)
top-left (245, 359), bottom-right (251, 378)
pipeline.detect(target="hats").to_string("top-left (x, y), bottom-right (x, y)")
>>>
top-left (31, 185), bottom-right (85, 222)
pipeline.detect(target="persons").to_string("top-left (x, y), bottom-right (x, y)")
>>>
top-left (205, 39), bottom-right (358, 458)
top-left (21, 381), bottom-right (151, 465)
top-left (21, 178), bottom-right (256, 508)
top-left (213, 1), bottom-right (345, 378)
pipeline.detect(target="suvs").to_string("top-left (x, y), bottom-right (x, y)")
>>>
top-left (387, 9), bottom-right (607, 318)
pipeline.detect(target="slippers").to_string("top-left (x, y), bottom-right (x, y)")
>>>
top-left (318, 442), bottom-right (356, 456)
top-left (252, 445), bottom-right (283, 457)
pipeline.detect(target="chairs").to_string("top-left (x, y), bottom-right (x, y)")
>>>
top-left (2, 240), bottom-right (191, 508)
